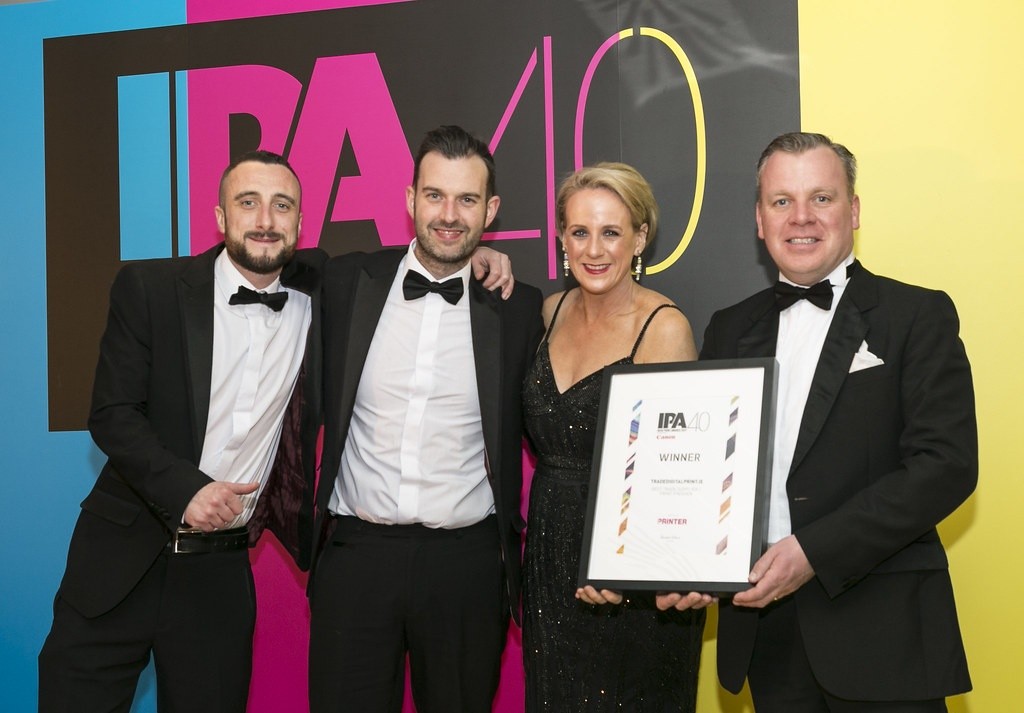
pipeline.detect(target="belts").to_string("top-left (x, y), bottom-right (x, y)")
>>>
top-left (165, 530), bottom-right (250, 554)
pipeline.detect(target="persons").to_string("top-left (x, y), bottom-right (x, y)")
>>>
top-left (655, 133), bottom-right (979, 713)
top-left (305, 123), bottom-right (544, 713)
top-left (518, 161), bottom-right (707, 713)
top-left (38, 149), bottom-right (514, 713)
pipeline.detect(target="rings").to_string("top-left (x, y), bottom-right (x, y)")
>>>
top-left (774, 597), bottom-right (777, 600)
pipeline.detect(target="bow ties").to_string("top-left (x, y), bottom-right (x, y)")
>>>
top-left (772, 279), bottom-right (834, 312)
top-left (228, 285), bottom-right (289, 312)
top-left (402, 269), bottom-right (464, 306)
